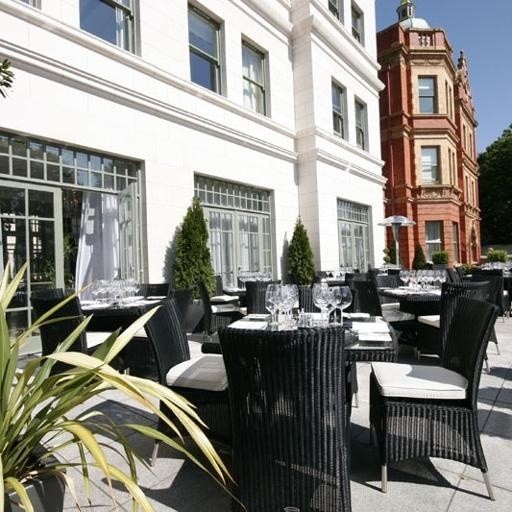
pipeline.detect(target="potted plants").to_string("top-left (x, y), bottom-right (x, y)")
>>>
top-left (169, 194), bottom-right (217, 333)
top-left (0, 259), bottom-right (247, 512)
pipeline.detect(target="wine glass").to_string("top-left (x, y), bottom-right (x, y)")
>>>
top-left (325, 266), bottom-right (353, 280)
top-left (238, 271), bottom-right (271, 290)
top-left (311, 282), bottom-right (353, 331)
top-left (88, 279), bottom-right (141, 306)
top-left (377, 265), bottom-right (398, 274)
top-left (265, 282), bottom-right (300, 330)
top-left (480, 261), bottom-right (512, 277)
top-left (399, 269), bottom-right (447, 293)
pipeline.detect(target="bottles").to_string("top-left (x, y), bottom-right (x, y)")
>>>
top-left (297, 313), bottom-right (312, 330)
